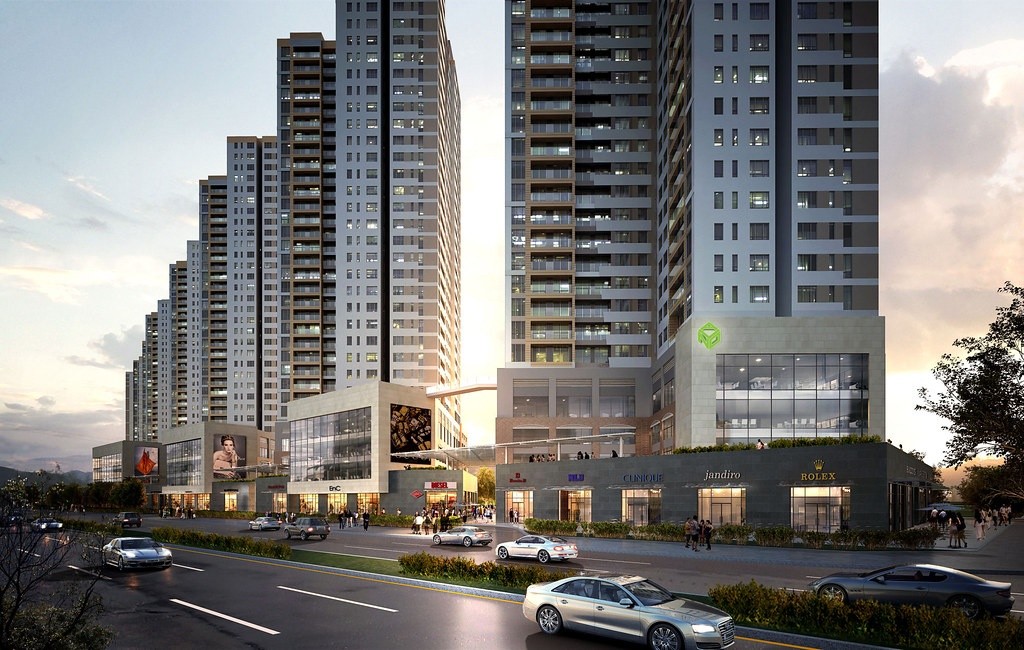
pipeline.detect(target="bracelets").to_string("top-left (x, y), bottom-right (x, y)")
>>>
top-left (231, 460), bottom-right (236, 463)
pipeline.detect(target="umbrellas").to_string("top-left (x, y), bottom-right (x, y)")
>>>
top-left (916, 503), bottom-right (965, 511)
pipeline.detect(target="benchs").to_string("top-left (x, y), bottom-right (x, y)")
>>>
top-left (568, 580), bottom-right (593, 597)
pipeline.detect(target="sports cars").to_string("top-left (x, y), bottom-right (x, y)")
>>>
top-left (805, 562), bottom-right (1016, 623)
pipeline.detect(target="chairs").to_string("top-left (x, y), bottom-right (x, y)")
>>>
top-left (601, 586), bottom-right (611, 601)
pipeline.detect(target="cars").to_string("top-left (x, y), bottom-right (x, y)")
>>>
top-left (524, 571), bottom-right (736, 650)
top-left (495, 535), bottom-right (579, 565)
top-left (102, 536), bottom-right (173, 573)
top-left (30, 517), bottom-right (64, 533)
top-left (5, 511), bottom-right (26, 526)
top-left (432, 524), bottom-right (494, 548)
top-left (248, 516), bottom-right (282, 532)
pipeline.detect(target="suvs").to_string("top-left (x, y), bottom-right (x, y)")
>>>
top-left (112, 511), bottom-right (143, 529)
top-left (282, 516), bottom-right (331, 542)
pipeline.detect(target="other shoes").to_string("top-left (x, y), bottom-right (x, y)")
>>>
top-left (688, 544), bottom-right (691, 546)
top-left (702, 544), bottom-right (705, 546)
top-left (700, 544), bottom-right (701, 546)
top-left (964, 542), bottom-right (968, 547)
top-left (706, 548), bottom-right (711, 551)
top-left (692, 548), bottom-right (695, 550)
top-left (685, 546), bottom-right (689, 548)
top-left (695, 550), bottom-right (700, 552)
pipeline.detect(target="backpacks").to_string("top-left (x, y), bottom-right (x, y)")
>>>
top-left (425, 516), bottom-right (430, 524)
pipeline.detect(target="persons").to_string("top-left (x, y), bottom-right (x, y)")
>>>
top-left (685, 515), bottom-right (714, 552)
top-left (158, 505), bottom-right (196, 520)
top-left (611, 449), bottom-right (618, 458)
top-left (577, 450), bottom-right (595, 460)
top-left (338, 507), bottom-right (401, 531)
top-left (529, 453), bottom-right (555, 463)
top-left (213, 435), bottom-right (241, 478)
top-left (974, 503), bottom-right (1012, 540)
top-left (411, 505), bottom-right (519, 536)
top-left (328, 503), bottom-right (334, 514)
top-left (927, 507), bottom-right (967, 548)
top-left (756, 439), bottom-right (764, 449)
top-left (265, 511), bottom-right (294, 524)
